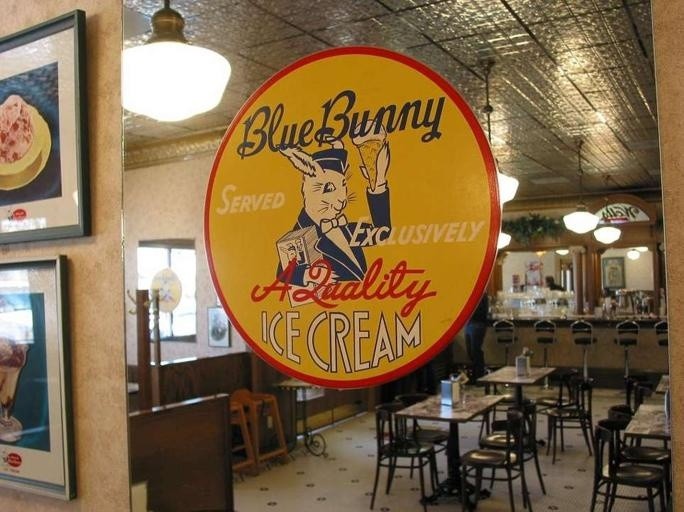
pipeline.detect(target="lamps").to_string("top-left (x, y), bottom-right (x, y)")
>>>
top-left (119, 0), bottom-right (231, 123)
top-left (562, 137), bottom-right (599, 233)
top-left (473, 58), bottom-right (518, 205)
top-left (596, 173), bottom-right (621, 248)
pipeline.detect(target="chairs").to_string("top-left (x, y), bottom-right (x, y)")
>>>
top-left (653, 319), bottom-right (668, 374)
top-left (570, 320), bottom-right (596, 384)
top-left (614, 319), bottom-right (640, 380)
top-left (534, 320), bottom-right (556, 390)
top-left (492, 319), bottom-right (516, 388)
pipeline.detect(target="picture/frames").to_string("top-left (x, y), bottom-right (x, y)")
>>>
top-left (0, 6), bottom-right (92, 251)
top-left (206, 306), bottom-right (234, 350)
top-left (0, 252), bottom-right (78, 502)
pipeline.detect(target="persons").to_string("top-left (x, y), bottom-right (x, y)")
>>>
top-left (541, 275), bottom-right (564, 303)
top-left (464, 292), bottom-right (490, 383)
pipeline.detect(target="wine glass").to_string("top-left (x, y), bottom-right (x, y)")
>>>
top-left (0, 349), bottom-right (28, 444)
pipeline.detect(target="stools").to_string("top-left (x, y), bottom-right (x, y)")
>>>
top-left (227, 390), bottom-right (289, 477)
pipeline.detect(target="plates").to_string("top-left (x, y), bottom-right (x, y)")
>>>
top-left (0, 102), bottom-right (43, 175)
top-left (0, 115), bottom-right (52, 191)
